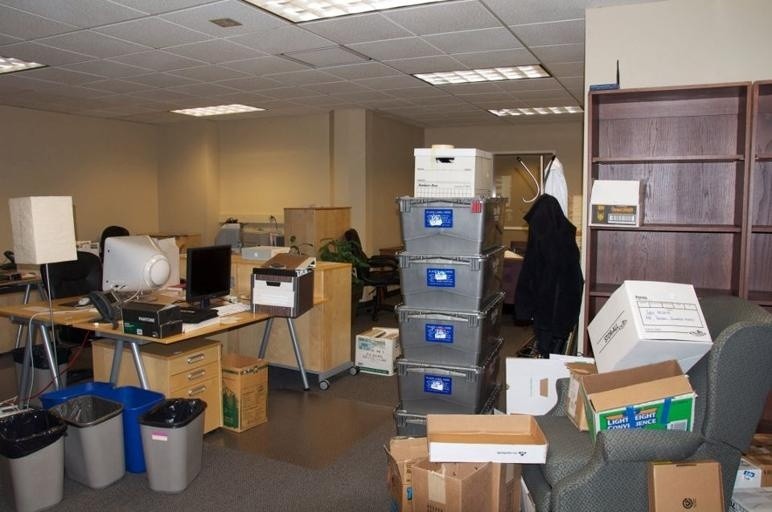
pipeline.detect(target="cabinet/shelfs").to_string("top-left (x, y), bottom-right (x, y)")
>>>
top-left (178, 253), bottom-right (354, 382)
top-left (91, 336), bottom-right (225, 438)
top-left (283, 207), bottom-right (353, 263)
top-left (746, 80), bottom-right (772, 318)
top-left (151, 232), bottom-right (202, 254)
top-left (582, 78), bottom-right (751, 361)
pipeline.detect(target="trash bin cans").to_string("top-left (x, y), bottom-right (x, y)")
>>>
top-left (48, 394), bottom-right (126, 490)
top-left (138, 397), bottom-right (208, 493)
top-left (0, 408), bottom-right (68, 512)
top-left (93, 385), bottom-right (166, 473)
top-left (38, 381), bottom-right (115, 412)
top-left (11, 343), bottom-right (69, 407)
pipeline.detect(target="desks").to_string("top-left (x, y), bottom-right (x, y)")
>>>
top-left (1, 293), bottom-right (171, 411)
top-left (73, 294), bottom-right (330, 393)
top-left (0, 269), bottom-right (49, 351)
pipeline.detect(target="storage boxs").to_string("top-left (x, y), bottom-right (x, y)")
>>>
top-left (355, 324), bottom-right (405, 378)
top-left (219, 352), bottom-right (270, 436)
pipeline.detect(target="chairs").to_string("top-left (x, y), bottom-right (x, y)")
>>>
top-left (342, 224), bottom-right (402, 325)
top-left (41, 251), bottom-right (104, 386)
top-left (98, 226), bottom-right (131, 259)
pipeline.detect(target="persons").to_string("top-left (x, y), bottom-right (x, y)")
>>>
top-left (81, 226), bottom-right (130, 296)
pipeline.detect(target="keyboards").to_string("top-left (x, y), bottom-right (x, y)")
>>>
top-left (209, 302), bottom-right (251, 317)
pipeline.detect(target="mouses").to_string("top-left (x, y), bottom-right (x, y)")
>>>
top-left (78, 298), bottom-right (90, 306)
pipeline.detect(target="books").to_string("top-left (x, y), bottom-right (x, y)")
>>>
top-left (180, 305), bottom-right (220, 333)
top-left (361, 327), bottom-right (399, 339)
top-left (253, 252), bottom-right (317, 278)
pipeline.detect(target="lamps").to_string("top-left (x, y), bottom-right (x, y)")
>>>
top-left (8, 195), bottom-right (84, 393)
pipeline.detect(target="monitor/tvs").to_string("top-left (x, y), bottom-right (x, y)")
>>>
top-left (102, 235), bottom-right (171, 292)
top-left (186, 243), bottom-right (231, 310)
